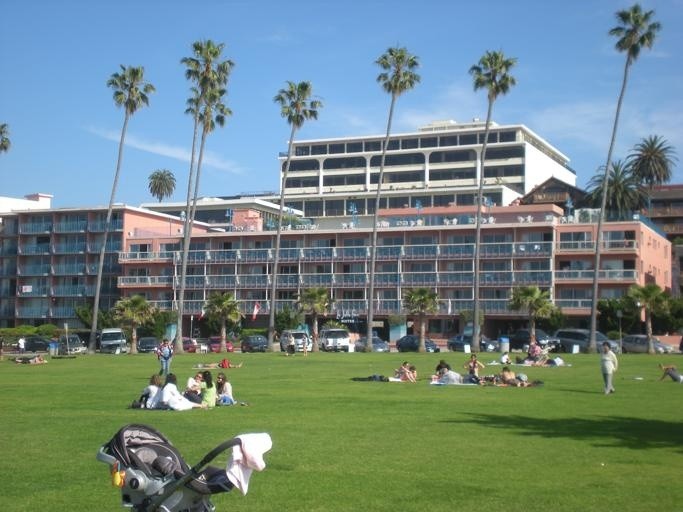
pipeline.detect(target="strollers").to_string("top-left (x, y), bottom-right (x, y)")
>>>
top-left (96, 423), bottom-right (272, 512)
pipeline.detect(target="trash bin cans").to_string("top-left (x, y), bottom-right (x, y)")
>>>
top-left (49, 342), bottom-right (59, 356)
top-left (497, 337), bottom-right (509, 353)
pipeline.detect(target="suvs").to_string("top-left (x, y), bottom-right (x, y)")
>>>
top-left (509, 328), bottom-right (561, 352)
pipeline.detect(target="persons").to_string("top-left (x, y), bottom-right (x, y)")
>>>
top-left (204, 359), bottom-right (244, 368)
top-left (129, 371), bottom-right (238, 412)
top-left (156, 338), bottom-right (173, 376)
top-left (15, 355), bottom-right (48, 365)
top-left (656, 363), bottom-right (683, 384)
top-left (16, 335), bottom-right (26, 355)
top-left (399, 340), bottom-right (565, 389)
top-left (601, 341), bottom-right (618, 395)
top-left (302, 334), bottom-right (308, 357)
top-left (285, 333), bottom-right (296, 357)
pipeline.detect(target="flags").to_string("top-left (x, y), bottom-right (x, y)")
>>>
top-left (251, 303), bottom-right (261, 321)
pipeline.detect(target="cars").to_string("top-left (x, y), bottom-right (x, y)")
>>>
top-left (354, 337), bottom-right (389, 353)
top-left (395, 335), bottom-right (439, 353)
top-left (171, 336), bottom-right (196, 353)
top-left (137, 337), bottom-right (158, 353)
top-left (208, 336), bottom-right (233, 352)
top-left (59, 334), bottom-right (83, 356)
top-left (16, 337), bottom-right (57, 351)
top-left (446, 333), bottom-right (496, 353)
top-left (622, 334), bottom-right (672, 354)
top-left (241, 335), bottom-right (266, 351)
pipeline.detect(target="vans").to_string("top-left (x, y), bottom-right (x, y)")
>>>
top-left (279, 330), bottom-right (310, 352)
top-left (553, 328), bottom-right (619, 354)
top-left (98, 328), bottom-right (127, 353)
top-left (319, 328), bottom-right (349, 352)
